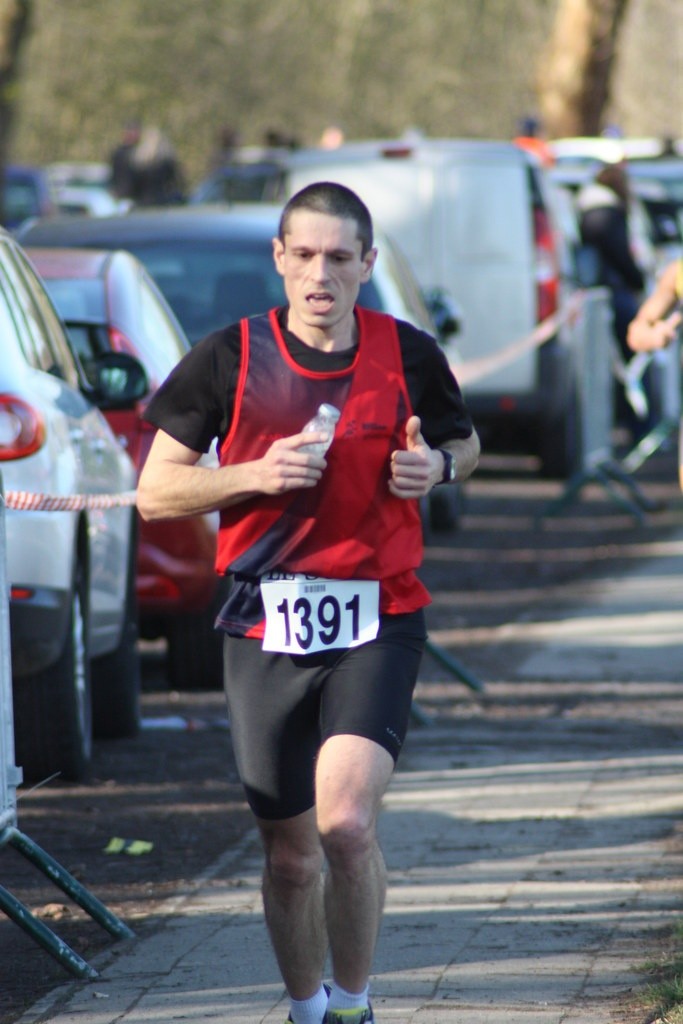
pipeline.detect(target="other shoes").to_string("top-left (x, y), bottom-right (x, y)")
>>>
top-left (285, 984), bottom-right (332, 1024)
top-left (322, 999), bottom-right (374, 1024)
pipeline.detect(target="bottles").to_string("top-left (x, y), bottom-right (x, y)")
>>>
top-left (298, 403), bottom-right (341, 456)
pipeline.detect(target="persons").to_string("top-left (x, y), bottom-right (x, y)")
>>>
top-left (135, 181), bottom-right (485, 1024)
top-left (107, 116), bottom-right (683, 495)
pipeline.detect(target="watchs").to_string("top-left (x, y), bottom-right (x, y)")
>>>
top-left (427, 446), bottom-right (455, 487)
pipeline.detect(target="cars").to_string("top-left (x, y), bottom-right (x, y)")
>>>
top-left (21, 245), bottom-right (225, 673)
top-left (0, 124), bottom-right (683, 474)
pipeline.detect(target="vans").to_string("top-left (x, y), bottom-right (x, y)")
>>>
top-left (0, 222), bottom-right (140, 780)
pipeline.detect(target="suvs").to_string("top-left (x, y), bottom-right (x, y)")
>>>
top-left (12, 196), bottom-right (468, 551)
